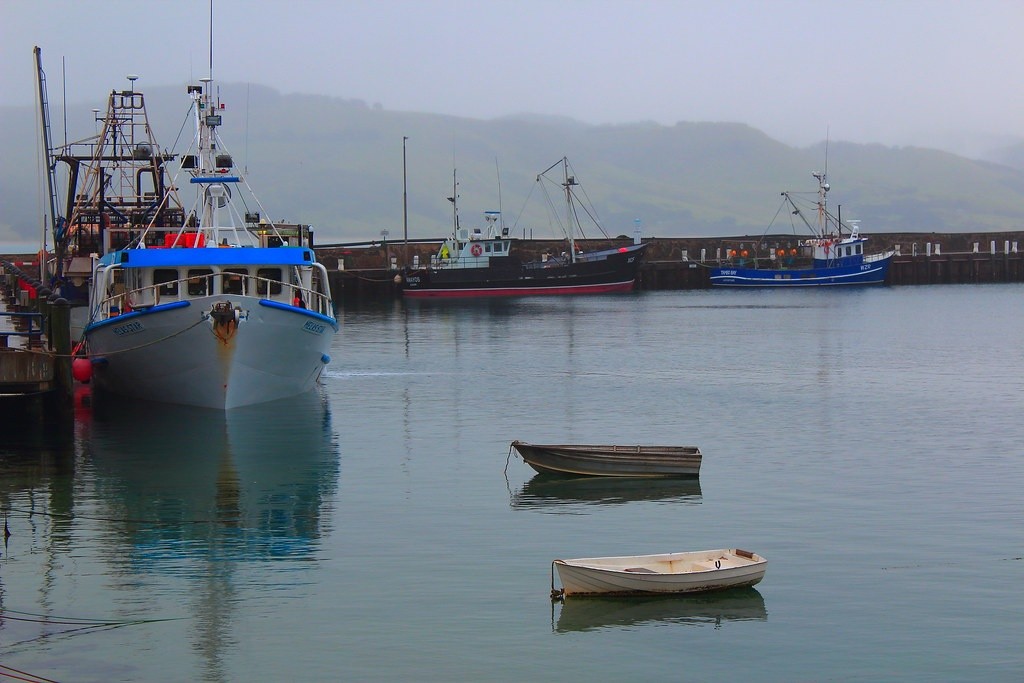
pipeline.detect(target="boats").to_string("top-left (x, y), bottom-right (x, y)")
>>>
top-left (511, 474), bottom-right (703, 507)
top-left (84, 78), bottom-right (339, 411)
top-left (47, 75), bottom-right (240, 347)
top-left (710, 172), bottom-right (897, 289)
top-left (511, 440), bottom-right (702, 478)
top-left (554, 549), bottom-right (767, 597)
top-left (556, 586), bottom-right (768, 632)
top-left (335, 156), bottom-right (648, 298)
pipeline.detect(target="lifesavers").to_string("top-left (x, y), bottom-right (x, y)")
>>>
top-left (471, 244), bottom-right (482, 257)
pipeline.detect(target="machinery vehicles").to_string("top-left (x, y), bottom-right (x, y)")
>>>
top-left (34, 46), bottom-right (59, 254)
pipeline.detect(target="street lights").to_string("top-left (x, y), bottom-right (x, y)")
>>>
top-left (403, 136), bottom-right (408, 267)
